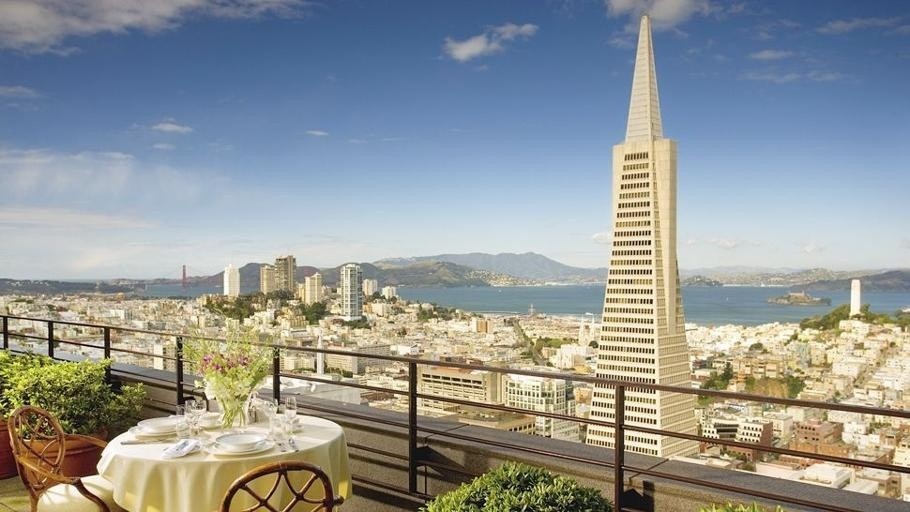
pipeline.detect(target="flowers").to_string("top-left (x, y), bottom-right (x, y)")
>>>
top-left (177, 320), bottom-right (290, 429)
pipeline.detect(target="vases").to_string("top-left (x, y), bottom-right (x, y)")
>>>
top-left (213, 389), bottom-right (254, 431)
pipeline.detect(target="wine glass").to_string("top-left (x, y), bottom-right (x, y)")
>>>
top-left (120, 394), bottom-right (302, 458)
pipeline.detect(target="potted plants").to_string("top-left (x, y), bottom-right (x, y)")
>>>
top-left (0, 347), bottom-right (150, 480)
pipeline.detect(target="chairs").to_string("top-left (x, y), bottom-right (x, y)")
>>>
top-left (217, 460), bottom-right (344, 511)
top-left (7, 405), bottom-right (118, 511)
